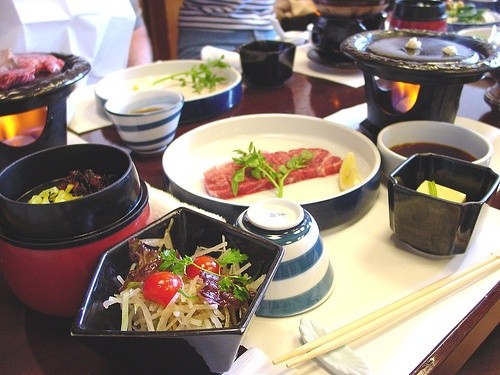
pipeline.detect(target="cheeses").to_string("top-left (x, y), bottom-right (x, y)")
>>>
top-left (415, 179), bottom-right (467, 204)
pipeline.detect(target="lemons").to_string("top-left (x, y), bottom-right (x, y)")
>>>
top-left (338, 150), bottom-right (362, 191)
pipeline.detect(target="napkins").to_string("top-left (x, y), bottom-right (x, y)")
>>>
top-left (201, 45), bottom-right (246, 74)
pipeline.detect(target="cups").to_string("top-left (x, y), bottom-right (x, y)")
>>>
top-left (387, 152), bottom-right (500, 257)
top-left (241, 38), bottom-right (295, 89)
top-left (104, 85), bottom-right (183, 154)
top-left (232, 199), bottom-right (335, 319)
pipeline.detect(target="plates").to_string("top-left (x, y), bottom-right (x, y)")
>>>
top-left (93, 57), bottom-right (244, 124)
top-left (160, 113), bottom-right (384, 238)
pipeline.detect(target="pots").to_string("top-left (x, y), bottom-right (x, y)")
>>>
top-left (311, 0), bottom-right (388, 17)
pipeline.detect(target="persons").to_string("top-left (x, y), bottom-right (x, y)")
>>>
top-left (127, 0), bottom-right (153, 68)
top-left (177, 0), bottom-right (277, 59)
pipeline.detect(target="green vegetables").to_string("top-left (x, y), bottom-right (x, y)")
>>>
top-left (28, 184), bottom-right (81, 204)
top-left (445, 3), bottom-right (485, 23)
top-left (231, 141), bottom-right (313, 198)
top-left (150, 54), bottom-right (229, 94)
top-left (159, 247), bottom-right (250, 301)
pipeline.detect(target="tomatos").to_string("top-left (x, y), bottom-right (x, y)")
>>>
top-left (142, 271), bottom-right (183, 306)
top-left (186, 256), bottom-right (224, 279)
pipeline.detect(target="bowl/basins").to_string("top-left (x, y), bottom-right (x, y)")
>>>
top-left (388, 0), bottom-right (447, 38)
top-left (440, 0), bottom-right (500, 55)
top-left (0, 143), bottom-right (153, 322)
top-left (69, 204), bottom-right (285, 375)
top-left (377, 120), bottom-right (494, 192)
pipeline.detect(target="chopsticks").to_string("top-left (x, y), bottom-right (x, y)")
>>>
top-left (273, 253), bottom-right (500, 366)
